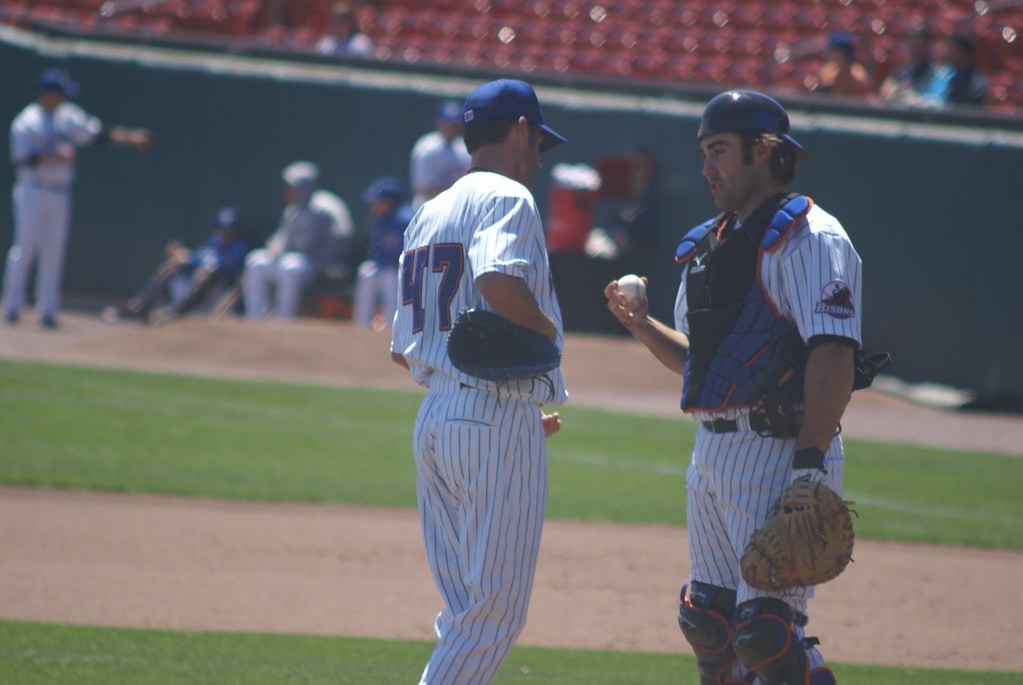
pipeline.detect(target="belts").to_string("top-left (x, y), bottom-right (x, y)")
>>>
top-left (702, 411), bottom-right (803, 433)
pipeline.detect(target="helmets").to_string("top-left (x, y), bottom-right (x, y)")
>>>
top-left (283, 161), bottom-right (320, 188)
top-left (697, 89), bottom-right (808, 161)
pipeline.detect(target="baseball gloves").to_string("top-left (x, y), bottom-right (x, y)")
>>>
top-left (444, 306), bottom-right (562, 408)
top-left (736, 471), bottom-right (860, 593)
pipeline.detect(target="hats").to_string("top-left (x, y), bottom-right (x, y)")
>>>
top-left (361, 177), bottom-right (405, 204)
top-left (462, 78), bottom-right (567, 153)
top-left (36, 67), bottom-right (76, 96)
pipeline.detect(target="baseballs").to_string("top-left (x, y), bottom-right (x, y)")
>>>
top-left (616, 273), bottom-right (647, 304)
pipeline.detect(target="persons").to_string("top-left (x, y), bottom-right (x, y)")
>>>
top-left (605, 90), bottom-right (863, 685)
top-left (100, 101), bottom-right (472, 328)
top-left (814, 33), bottom-right (987, 109)
top-left (316, 5), bottom-right (376, 60)
top-left (3, 67), bottom-right (149, 330)
top-left (391, 77), bottom-right (569, 685)
